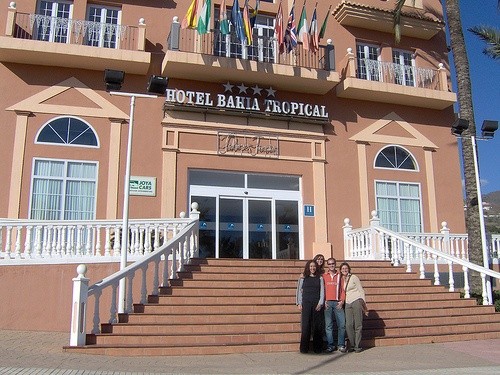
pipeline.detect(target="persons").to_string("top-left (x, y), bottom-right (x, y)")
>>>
top-left (299, 254), bottom-right (326, 337)
top-left (339, 263), bottom-right (367, 353)
top-left (296, 259), bottom-right (325, 353)
top-left (322, 258), bottom-right (347, 353)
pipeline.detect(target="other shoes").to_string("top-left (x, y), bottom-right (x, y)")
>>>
top-left (348, 349), bottom-right (361, 353)
top-left (339, 346), bottom-right (347, 353)
top-left (325, 347), bottom-right (334, 353)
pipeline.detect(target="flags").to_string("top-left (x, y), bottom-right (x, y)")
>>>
top-left (217, 0), bottom-right (260, 46)
top-left (274, 2), bottom-right (330, 56)
top-left (181, 0), bottom-right (211, 34)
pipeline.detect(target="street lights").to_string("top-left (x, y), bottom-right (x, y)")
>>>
top-left (450, 119), bottom-right (500, 305)
top-left (103, 68), bottom-right (170, 314)
